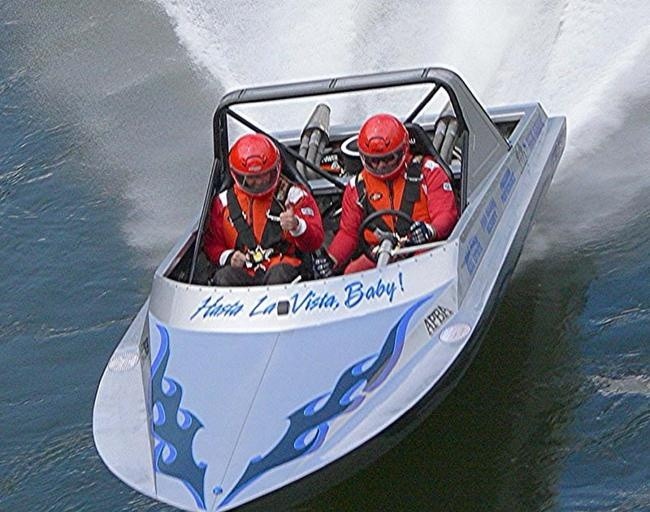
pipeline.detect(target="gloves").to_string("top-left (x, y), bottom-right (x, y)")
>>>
top-left (312, 256), bottom-right (335, 279)
top-left (408, 220), bottom-right (436, 244)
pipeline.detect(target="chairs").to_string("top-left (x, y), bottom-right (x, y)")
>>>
top-left (272, 122), bottom-right (456, 197)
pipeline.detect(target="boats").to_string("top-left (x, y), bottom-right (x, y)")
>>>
top-left (92, 67), bottom-right (568, 512)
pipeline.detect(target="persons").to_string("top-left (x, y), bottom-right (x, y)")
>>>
top-left (310, 112), bottom-right (459, 281)
top-left (203, 133), bottom-right (326, 288)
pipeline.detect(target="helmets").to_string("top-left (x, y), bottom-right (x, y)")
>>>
top-left (357, 113), bottom-right (410, 181)
top-left (228, 133), bottom-right (282, 202)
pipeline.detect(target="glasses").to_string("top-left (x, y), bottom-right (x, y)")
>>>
top-left (364, 155), bottom-right (398, 164)
